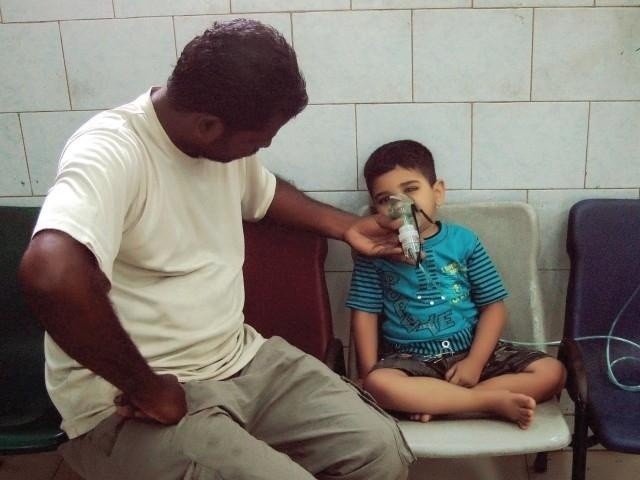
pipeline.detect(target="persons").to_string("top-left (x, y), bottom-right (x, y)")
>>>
top-left (344, 140), bottom-right (568, 430)
top-left (18, 18), bottom-right (418, 480)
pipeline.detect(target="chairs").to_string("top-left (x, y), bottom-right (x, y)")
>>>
top-left (353, 203), bottom-right (558, 474)
top-left (0, 206), bottom-right (64, 452)
top-left (556, 199), bottom-right (639, 480)
top-left (241, 219), bottom-right (346, 379)
